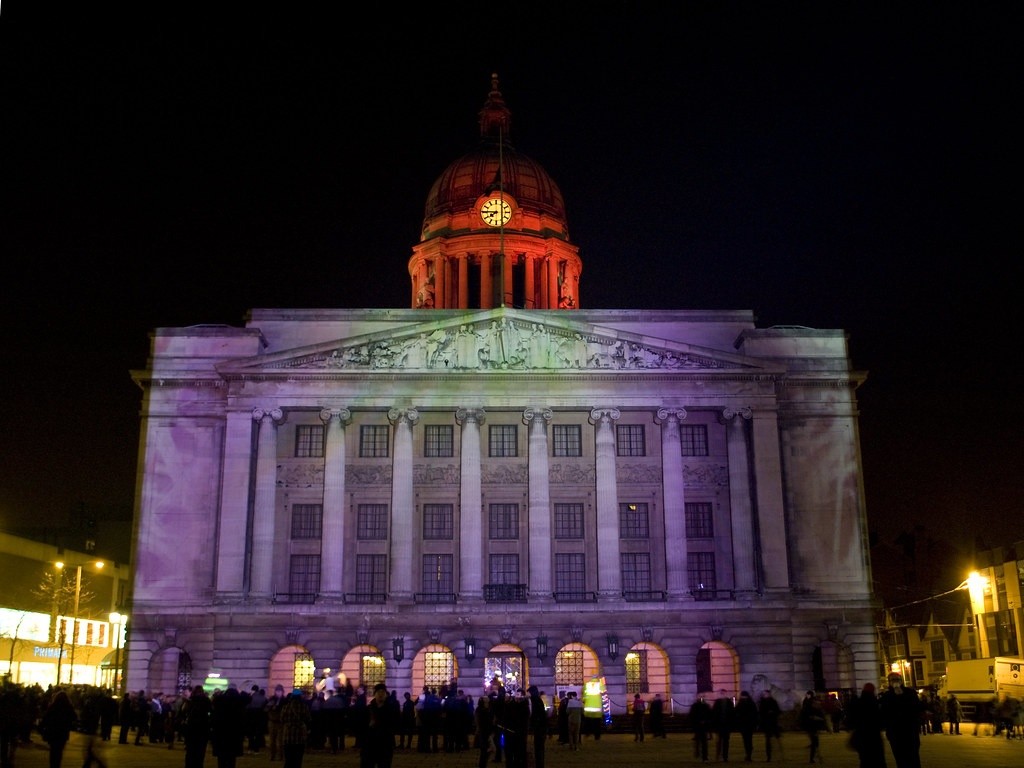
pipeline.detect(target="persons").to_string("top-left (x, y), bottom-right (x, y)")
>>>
top-left (880, 672), bottom-right (923, 768)
top-left (327, 317), bottom-right (645, 369)
top-left (921, 693), bottom-right (946, 735)
top-left (973, 696), bottom-right (1024, 740)
top-left (557, 690), bottom-right (584, 746)
top-left (689, 689), bottom-right (782, 763)
top-left (633, 694), bottom-right (646, 742)
top-left (850, 683), bottom-right (886, 768)
top-left (474, 686), bottom-right (553, 768)
top-left (651, 694), bottom-right (666, 739)
top-left (946, 695), bottom-right (962, 735)
top-left (800, 690), bottom-right (843, 763)
top-left (0, 673), bottom-right (475, 768)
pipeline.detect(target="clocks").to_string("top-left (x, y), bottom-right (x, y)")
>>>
top-left (479, 196), bottom-right (513, 228)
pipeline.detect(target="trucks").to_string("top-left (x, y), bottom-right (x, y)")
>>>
top-left (936, 656), bottom-right (1024, 721)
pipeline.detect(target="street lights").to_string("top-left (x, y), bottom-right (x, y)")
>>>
top-left (109, 612), bottom-right (128, 694)
top-left (56, 559), bottom-right (105, 686)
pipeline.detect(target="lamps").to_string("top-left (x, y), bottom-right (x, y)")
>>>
top-left (536, 625), bottom-right (547, 664)
top-left (463, 632), bottom-right (477, 663)
top-left (607, 625), bottom-right (619, 663)
top-left (392, 630), bottom-right (404, 666)
